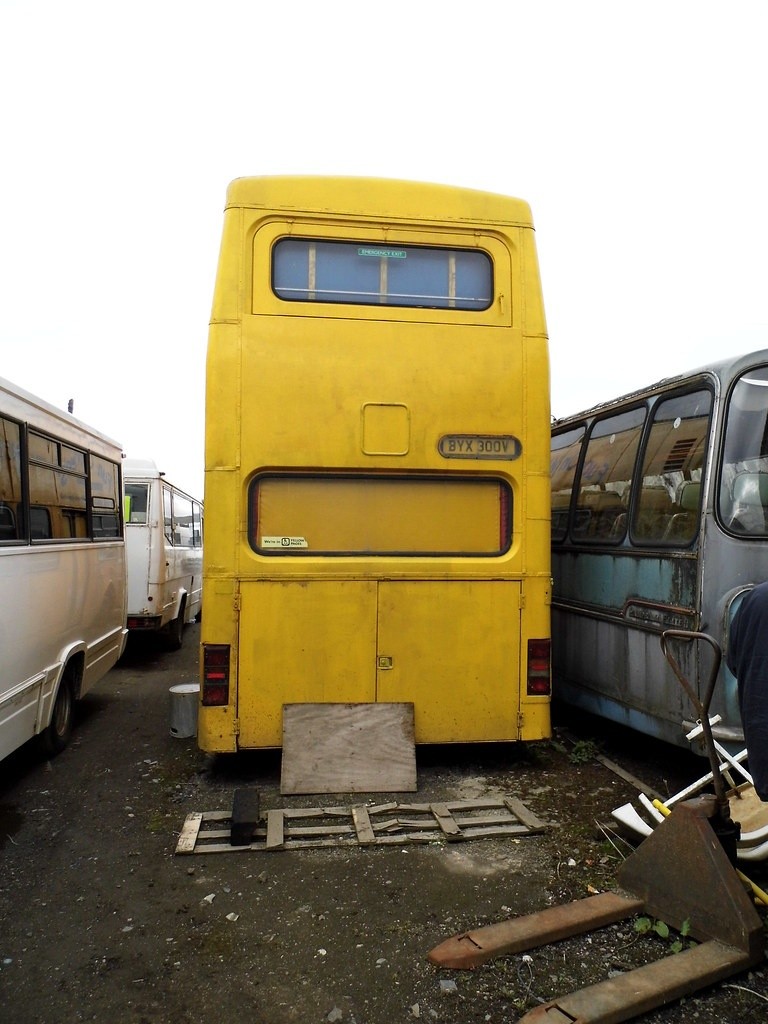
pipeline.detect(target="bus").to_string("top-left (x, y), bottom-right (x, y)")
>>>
top-left (0, 378), bottom-right (128, 764)
top-left (542, 350), bottom-right (768, 768)
top-left (121, 458), bottom-right (203, 649)
top-left (195, 175), bottom-right (555, 762)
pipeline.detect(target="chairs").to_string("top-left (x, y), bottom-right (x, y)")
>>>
top-left (551, 472), bottom-right (768, 544)
